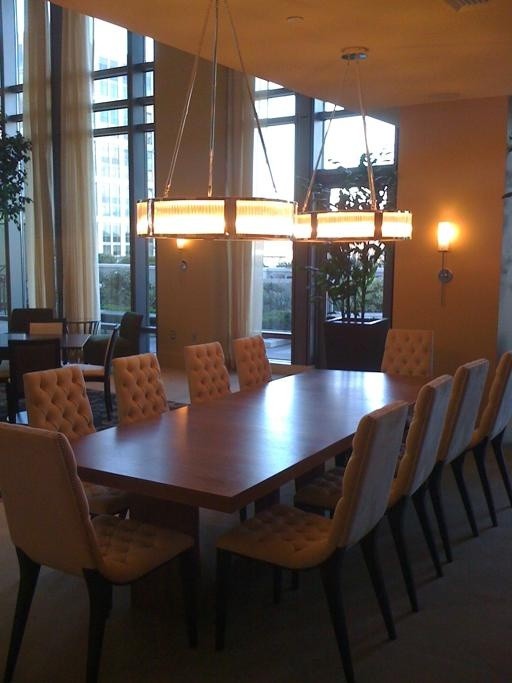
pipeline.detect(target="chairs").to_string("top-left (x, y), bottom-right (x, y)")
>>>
top-left (380, 328), bottom-right (435, 380)
top-left (6, 338), bottom-right (61, 423)
top-left (1, 421), bottom-right (200, 683)
top-left (111, 353), bottom-right (170, 425)
top-left (22, 365), bottom-right (131, 519)
top-left (217, 400), bottom-right (397, 683)
top-left (295, 375), bottom-right (452, 613)
top-left (472, 350), bottom-right (511, 528)
top-left (85, 311), bottom-right (144, 364)
top-left (183, 342), bottom-right (247, 523)
top-left (8, 307), bottom-right (54, 334)
top-left (428, 358), bottom-right (498, 536)
top-left (28, 318), bottom-right (68, 366)
top-left (233, 334), bottom-right (272, 390)
top-left (64, 323), bottom-right (121, 422)
top-left (66, 321), bottom-right (100, 364)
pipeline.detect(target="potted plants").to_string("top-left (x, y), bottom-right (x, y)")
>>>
top-left (295, 150), bottom-right (397, 371)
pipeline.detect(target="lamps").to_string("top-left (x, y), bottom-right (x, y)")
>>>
top-left (135, 0), bottom-right (298, 242)
top-left (298, 54), bottom-right (413, 242)
top-left (435, 221), bottom-right (461, 305)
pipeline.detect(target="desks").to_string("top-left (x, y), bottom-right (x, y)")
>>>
top-left (1, 332), bottom-right (91, 364)
top-left (71, 368), bottom-right (434, 636)
